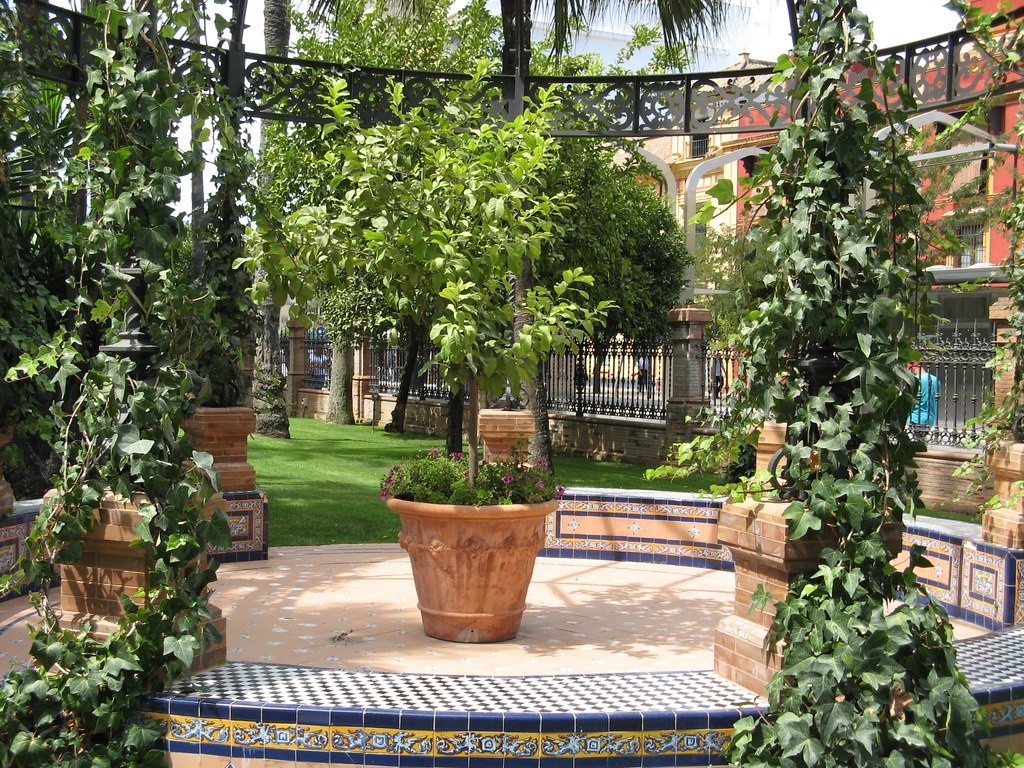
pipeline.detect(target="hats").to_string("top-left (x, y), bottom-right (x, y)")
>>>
top-left (906, 361), bottom-right (922, 370)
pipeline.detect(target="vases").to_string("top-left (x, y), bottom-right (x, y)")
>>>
top-left (385, 496), bottom-right (562, 644)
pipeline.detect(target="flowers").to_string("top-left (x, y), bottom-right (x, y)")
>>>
top-left (379, 448), bottom-right (566, 507)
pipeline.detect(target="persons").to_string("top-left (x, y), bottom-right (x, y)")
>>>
top-left (638, 357), bottom-right (650, 392)
top-left (710, 353), bottom-right (726, 399)
top-left (906, 361), bottom-right (941, 438)
top-left (309, 348), bottom-right (329, 388)
